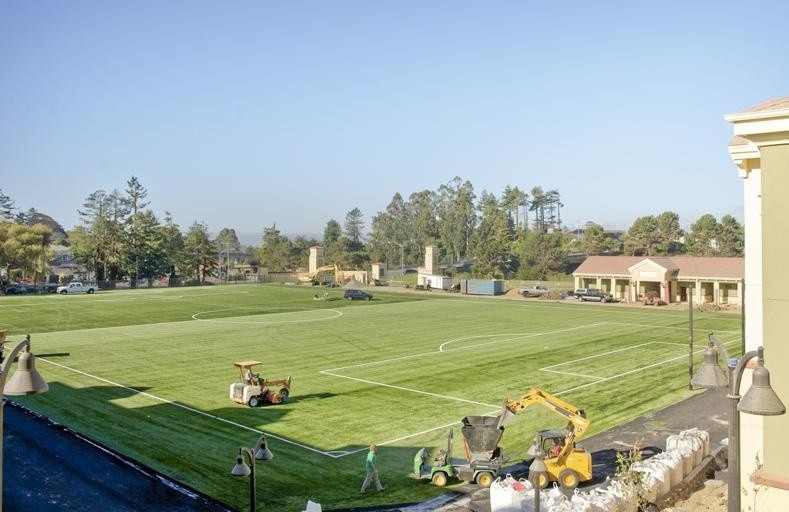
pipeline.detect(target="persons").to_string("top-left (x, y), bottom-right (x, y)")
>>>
top-left (358, 443), bottom-right (384, 494)
top-left (244, 369), bottom-right (255, 384)
top-left (549, 438), bottom-right (562, 457)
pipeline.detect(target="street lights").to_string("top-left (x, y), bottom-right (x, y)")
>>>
top-left (228, 432), bottom-right (277, 511)
top-left (387, 239), bottom-right (403, 289)
top-left (688, 328), bottom-right (788, 512)
top-left (218, 248), bottom-right (237, 281)
top-left (526, 430), bottom-right (550, 511)
top-left (0, 328), bottom-right (53, 511)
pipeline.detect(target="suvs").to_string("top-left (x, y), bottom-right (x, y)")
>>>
top-left (573, 287), bottom-right (614, 303)
top-left (343, 289), bottom-right (375, 302)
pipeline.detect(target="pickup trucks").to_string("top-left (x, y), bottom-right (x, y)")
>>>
top-left (518, 285), bottom-right (553, 298)
top-left (56, 282), bottom-right (99, 295)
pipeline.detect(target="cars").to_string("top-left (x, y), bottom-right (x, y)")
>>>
top-left (0, 281), bottom-right (34, 295)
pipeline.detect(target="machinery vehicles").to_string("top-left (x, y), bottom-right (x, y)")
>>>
top-left (459, 385), bottom-right (595, 493)
top-left (229, 360), bottom-right (293, 408)
